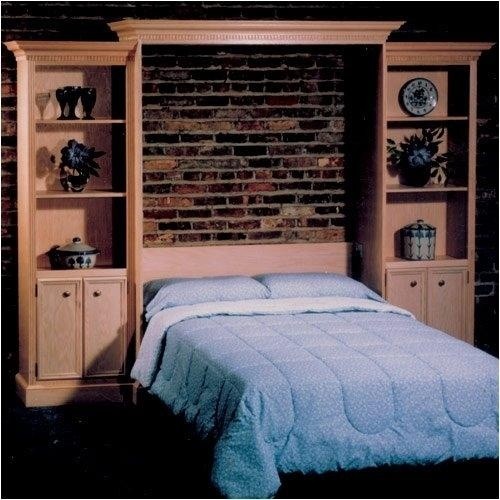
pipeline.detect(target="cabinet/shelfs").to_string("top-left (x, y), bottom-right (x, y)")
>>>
top-left (3, 41), bottom-right (144, 409)
top-left (371, 41), bottom-right (494, 346)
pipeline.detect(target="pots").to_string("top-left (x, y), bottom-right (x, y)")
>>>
top-left (47, 235), bottom-right (102, 268)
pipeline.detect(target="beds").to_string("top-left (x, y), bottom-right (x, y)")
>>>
top-left (130, 242), bottom-right (499, 498)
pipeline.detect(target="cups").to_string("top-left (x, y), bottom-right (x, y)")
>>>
top-left (401, 218), bottom-right (436, 261)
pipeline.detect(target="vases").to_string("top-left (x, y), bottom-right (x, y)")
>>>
top-left (399, 166), bottom-right (431, 187)
top-left (62, 176), bottom-right (87, 192)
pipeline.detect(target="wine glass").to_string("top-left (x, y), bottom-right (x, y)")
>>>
top-left (55, 84), bottom-right (97, 119)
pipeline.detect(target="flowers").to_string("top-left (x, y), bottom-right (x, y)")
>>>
top-left (387, 127), bottom-right (465, 187)
top-left (60, 140), bottom-right (106, 182)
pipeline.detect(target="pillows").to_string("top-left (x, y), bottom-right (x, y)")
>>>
top-left (146, 274), bottom-right (272, 323)
top-left (251, 271), bottom-right (387, 303)
